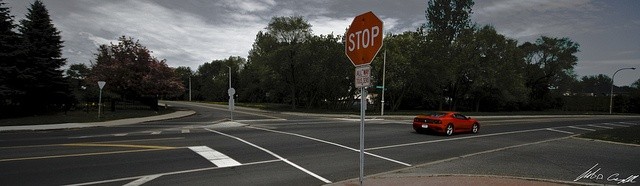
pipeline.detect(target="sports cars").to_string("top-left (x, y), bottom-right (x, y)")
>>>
top-left (413, 111), bottom-right (481, 135)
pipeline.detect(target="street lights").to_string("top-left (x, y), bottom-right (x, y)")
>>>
top-left (609, 66), bottom-right (635, 115)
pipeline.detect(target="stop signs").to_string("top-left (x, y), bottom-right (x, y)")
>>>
top-left (346, 11), bottom-right (383, 66)
